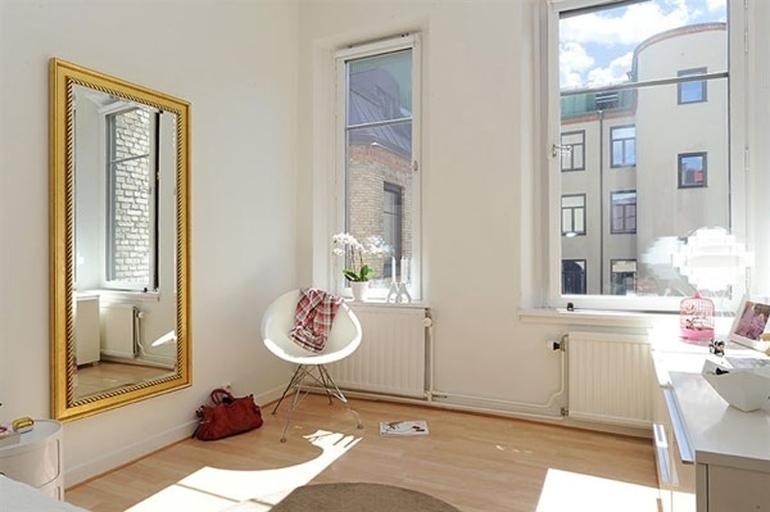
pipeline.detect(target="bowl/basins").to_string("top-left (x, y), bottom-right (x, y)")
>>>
top-left (701, 359), bottom-right (770, 413)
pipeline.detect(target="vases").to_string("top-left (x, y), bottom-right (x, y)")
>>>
top-left (350, 282), bottom-right (369, 302)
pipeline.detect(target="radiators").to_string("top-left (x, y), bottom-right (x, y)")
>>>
top-left (100, 302), bottom-right (144, 358)
top-left (567, 331), bottom-right (666, 431)
top-left (298, 302), bottom-right (426, 399)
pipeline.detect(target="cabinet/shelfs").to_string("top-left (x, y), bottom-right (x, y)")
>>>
top-left (651, 332), bottom-right (770, 512)
top-left (73, 291), bottom-right (100, 368)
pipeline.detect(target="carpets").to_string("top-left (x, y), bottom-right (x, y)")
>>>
top-left (220, 483), bottom-right (462, 512)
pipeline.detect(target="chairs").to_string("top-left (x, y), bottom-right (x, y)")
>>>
top-left (259, 288), bottom-right (364, 443)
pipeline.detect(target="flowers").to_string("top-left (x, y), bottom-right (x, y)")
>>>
top-left (331, 232), bottom-right (396, 282)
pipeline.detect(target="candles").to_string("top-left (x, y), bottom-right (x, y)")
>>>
top-left (400, 255), bottom-right (405, 282)
top-left (392, 256), bottom-right (396, 282)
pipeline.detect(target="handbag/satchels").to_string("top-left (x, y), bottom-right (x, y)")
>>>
top-left (195, 389), bottom-right (263, 441)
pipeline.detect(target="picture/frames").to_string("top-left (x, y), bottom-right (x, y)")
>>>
top-left (728, 293), bottom-right (770, 352)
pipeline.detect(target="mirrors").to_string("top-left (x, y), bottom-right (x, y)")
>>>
top-left (48, 56), bottom-right (192, 424)
